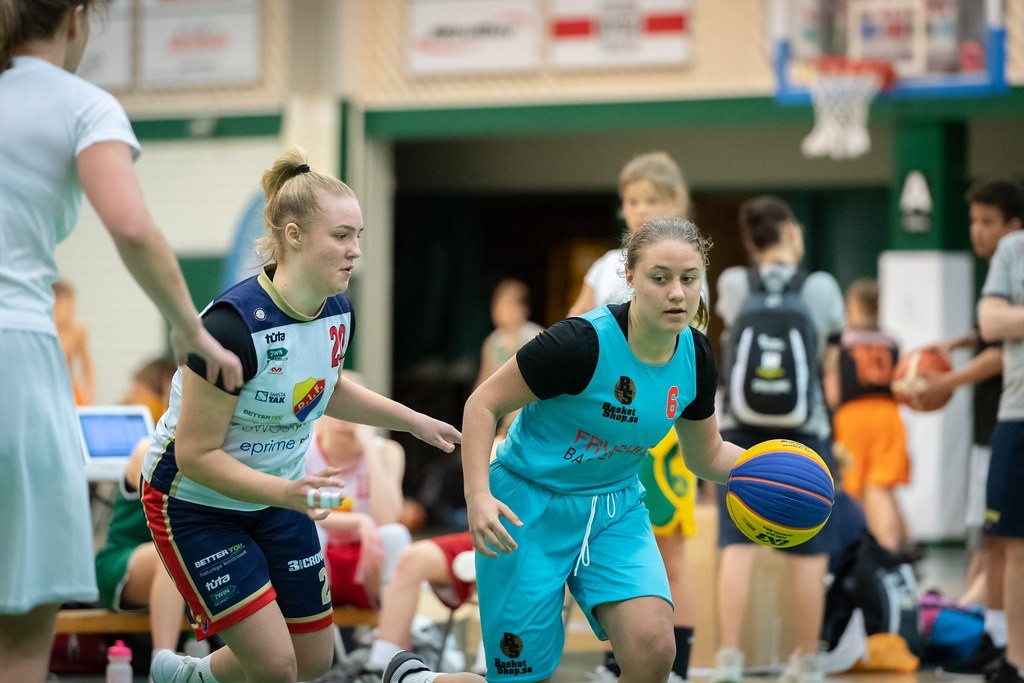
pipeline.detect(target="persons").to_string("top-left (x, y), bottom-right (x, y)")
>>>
top-left (472, 282), bottom-right (547, 393)
top-left (825, 279), bottom-right (911, 553)
top-left (568, 151), bottom-right (709, 683)
top-left (94, 438), bottom-right (184, 653)
top-left (51, 279), bottom-right (94, 402)
top-left (382, 217), bottom-right (747, 683)
top-left (0, 1), bottom-right (244, 683)
top-left (979, 228), bottom-right (1024, 683)
top-left (821, 452), bottom-right (917, 671)
top-left (124, 359), bottom-right (178, 424)
top-left (137, 146), bottom-right (464, 683)
top-left (344, 531), bottom-right (488, 683)
top-left (893, 175), bottom-right (1024, 612)
top-left (301, 369), bottom-right (420, 603)
top-left (717, 197), bottom-right (847, 683)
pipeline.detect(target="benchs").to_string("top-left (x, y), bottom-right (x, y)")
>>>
top-left (54, 607), bottom-right (379, 659)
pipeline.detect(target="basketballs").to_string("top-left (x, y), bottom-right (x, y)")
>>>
top-left (724, 439), bottom-right (835, 551)
top-left (888, 345), bottom-right (958, 413)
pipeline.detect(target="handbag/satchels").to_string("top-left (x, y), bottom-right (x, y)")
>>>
top-left (917, 589), bottom-right (957, 635)
top-left (928, 606), bottom-right (1004, 675)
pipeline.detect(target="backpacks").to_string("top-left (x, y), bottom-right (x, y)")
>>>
top-left (723, 261), bottom-right (820, 430)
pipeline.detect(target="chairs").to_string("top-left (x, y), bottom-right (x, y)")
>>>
top-left (435, 590), bottom-right (575, 672)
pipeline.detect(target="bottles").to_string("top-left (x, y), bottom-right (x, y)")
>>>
top-left (105, 641), bottom-right (133, 683)
top-left (184, 630), bottom-right (211, 659)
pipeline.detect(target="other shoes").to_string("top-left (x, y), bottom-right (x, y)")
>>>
top-left (935, 632), bottom-right (1007, 683)
top-left (714, 649), bottom-right (743, 683)
top-left (342, 666), bottom-right (383, 683)
top-left (778, 647), bottom-right (826, 683)
top-left (983, 656), bottom-right (1024, 683)
top-left (597, 650), bottom-right (621, 683)
top-left (150, 649), bottom-right (201, 683)
top-left (381, 649), bottom-right (431, 683)
